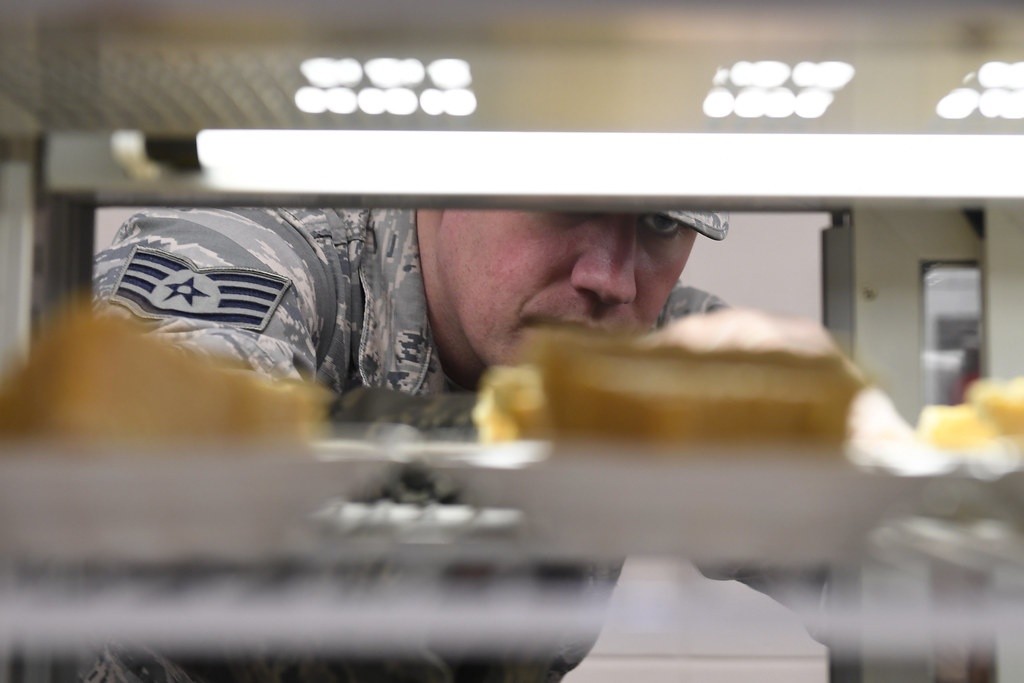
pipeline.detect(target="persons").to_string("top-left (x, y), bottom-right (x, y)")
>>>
top-left (94, 205), bottom-right (728, 683)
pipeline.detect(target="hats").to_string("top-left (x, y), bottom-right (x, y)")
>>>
top-left (663, 208), bottom-right (732, 241)
